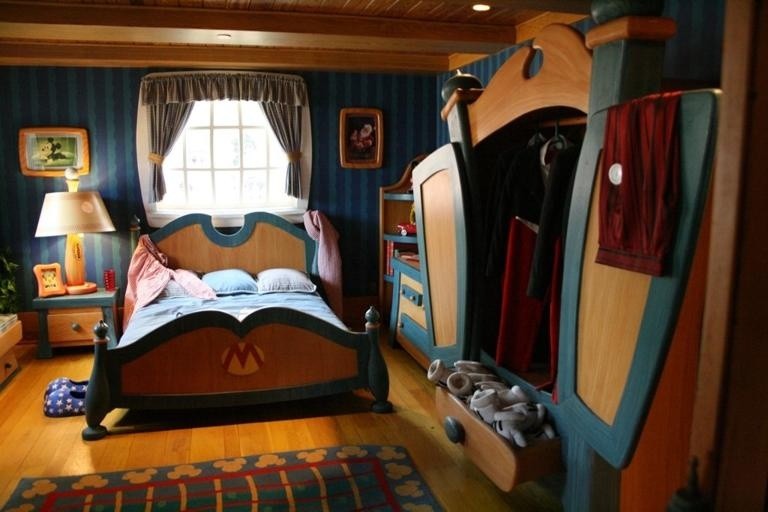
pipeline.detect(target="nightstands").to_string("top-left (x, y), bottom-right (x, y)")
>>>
top-left (32, 288), bottom-right (118, 358)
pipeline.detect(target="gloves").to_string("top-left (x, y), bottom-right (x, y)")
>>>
top-left (427, 359), bottom-right (555, 447)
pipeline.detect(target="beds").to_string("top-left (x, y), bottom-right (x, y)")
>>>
top-left (81, 214), bottom-right (392, 441)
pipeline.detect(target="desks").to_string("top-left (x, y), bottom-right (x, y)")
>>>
top-left (0, 313), bottom-right (23, 384)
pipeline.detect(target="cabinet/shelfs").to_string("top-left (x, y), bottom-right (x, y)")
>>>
top-left (379, 153), bottom-right (432, 371)
top-left (412, 1), bottom-right (768, 511)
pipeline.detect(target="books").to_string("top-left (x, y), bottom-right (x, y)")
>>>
top-left (385, 241), bottom-right (418, 277)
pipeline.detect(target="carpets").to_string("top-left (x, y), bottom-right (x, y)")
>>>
top-left (0, 446), bottom-right (447, 512)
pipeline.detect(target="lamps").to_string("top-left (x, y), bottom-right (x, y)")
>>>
top-left (34, 167), bottom-right (115, 295)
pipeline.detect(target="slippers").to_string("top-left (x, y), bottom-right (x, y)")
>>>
top-left (43, 377), bottom-right (88, 417)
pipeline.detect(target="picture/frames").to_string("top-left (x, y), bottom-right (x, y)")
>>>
top-left (339, 108), bottom-right (385, 169)
top-left (33, 263), bottom-right (66, 297)
top-left (19, 127), bottom-right (90, 176)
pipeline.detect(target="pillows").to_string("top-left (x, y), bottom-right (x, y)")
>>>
top-left (155, 279), bottom-right (197, 300)
top-left (254, 268), bottom-right (318, 296)
top-left (202, 268), bottom-right (258, 296)
